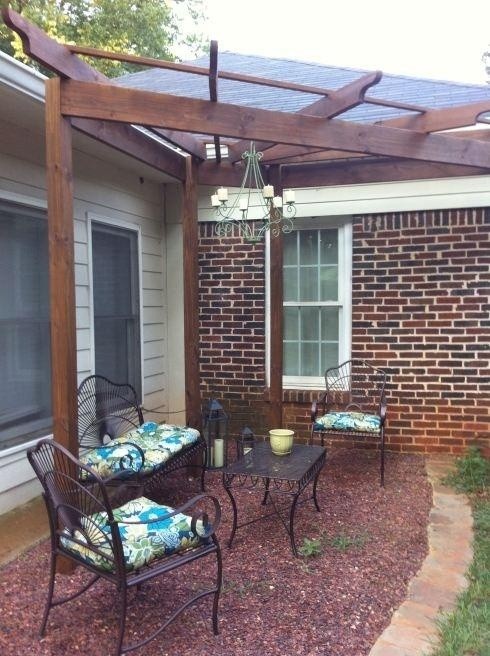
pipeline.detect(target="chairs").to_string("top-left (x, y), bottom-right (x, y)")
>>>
top-left (309, 358), bottom-right (388, 486)
top-left (26, 439), bottom-right (223, 656)
top-left (61, 375), bottom-right (209, 496)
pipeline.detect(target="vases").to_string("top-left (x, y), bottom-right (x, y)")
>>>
top-left (269, 428), bottom-right (295, 455)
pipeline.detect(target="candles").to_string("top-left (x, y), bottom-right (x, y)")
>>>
top-left (240, 447), bottom-right (253, 469)
top-left (285, 188), bottom-right (295, 201)
top-left (263, 184), bottom-right (274, 197)
top-left (272, 195), bottom-right (283, 209)
top-left (239, 197), bottom-right (248, 210)
top-left (210, 192), bottom-right (222, 206)
top-left (216, 186), bottom-right (228, 200)
top-left (214, 438), bottom-right (224, 467)
